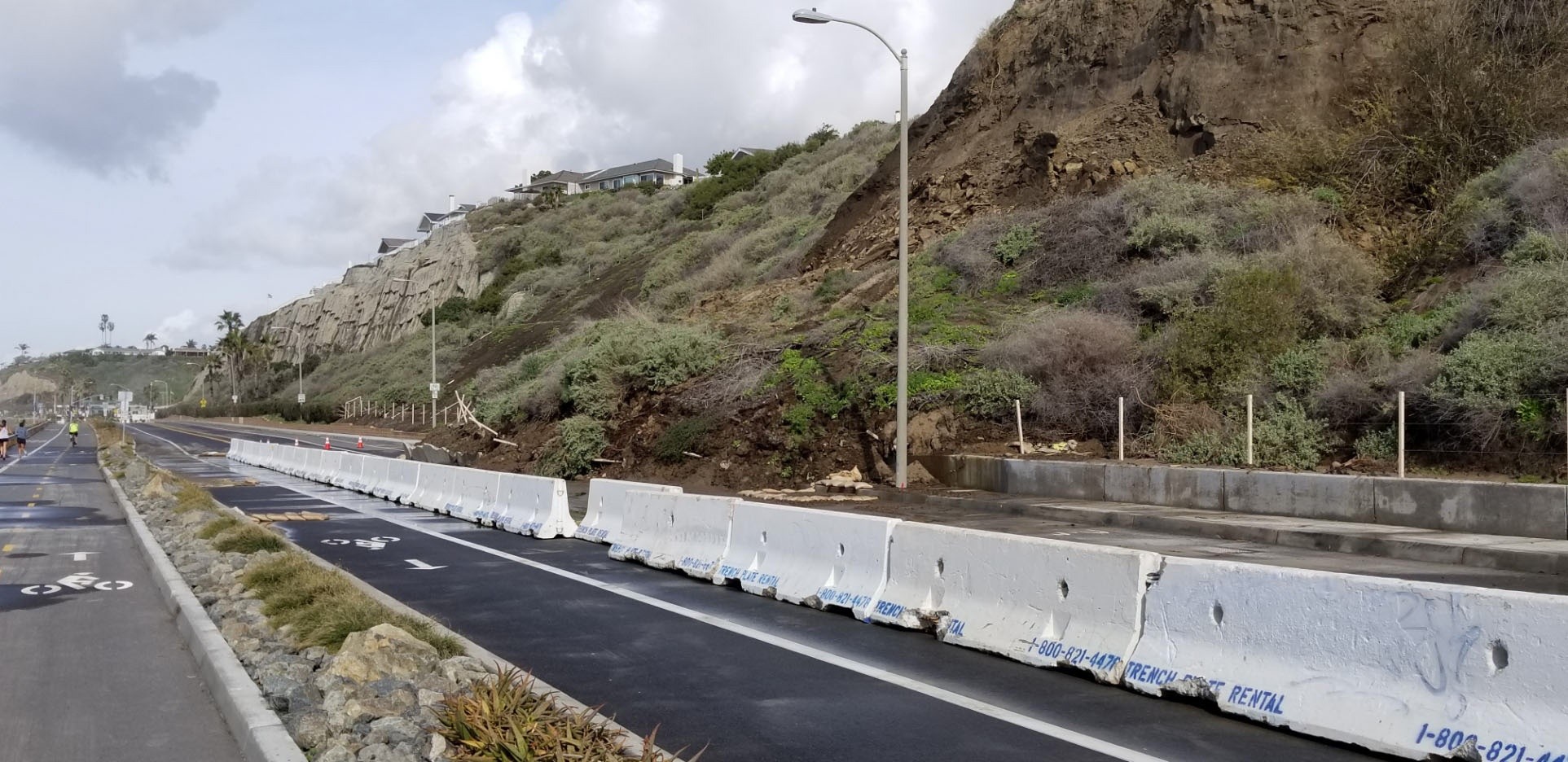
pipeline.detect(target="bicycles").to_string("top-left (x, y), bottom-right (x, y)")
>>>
top-left (69, 431), bottom-right (79, 448)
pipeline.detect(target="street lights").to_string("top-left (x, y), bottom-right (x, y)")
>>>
top-left (32, 383), bottom-right (55, 416)
top-left (186, 362), bottom-right (204, 404)
top-left (270, 325), bottom-right (303, 423)
top-left (70, 381), bottom-right (93, 407)
top-left (392, 278), bottom-right (437, 427)
top-left (155, 380), bottom-right (168, 408)
top-left (110, 384), bottom-right (129, 393)
top-left (791, 6), bottom-right (909, 491)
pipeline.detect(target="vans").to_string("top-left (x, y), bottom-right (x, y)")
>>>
top-left (55, 414), bottom-right (64, 425)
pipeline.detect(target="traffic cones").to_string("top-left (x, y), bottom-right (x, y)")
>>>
top-left (356, 434), bottom-right (364, 448)
top-left (322, 436), bottom-right (332, 450)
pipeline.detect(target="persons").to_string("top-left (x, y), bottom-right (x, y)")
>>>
top-left (17, 423), bottom-right (27, 458)
top-left (0, 420), bottom-right (9, 461)
top-left (69, 421), bottom-right (78, 444)
top-left (200, 398), bottom-right (207, 410)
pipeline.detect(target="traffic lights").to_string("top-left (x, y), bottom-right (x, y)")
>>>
top-left (104, 396), bottom-right (105, 399)
top-left (109, 395), bottom-right (112, 401)
top-left (97, 396), bottom-right (100, 401)
